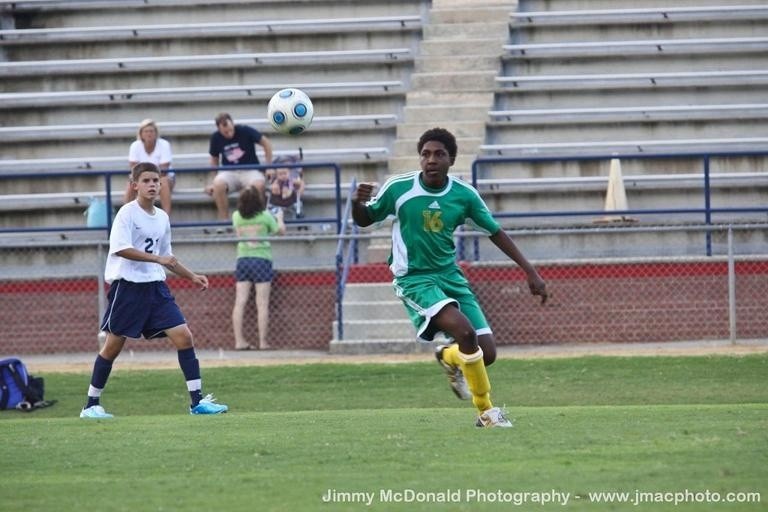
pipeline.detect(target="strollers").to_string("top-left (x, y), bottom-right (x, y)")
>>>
top-left (265, 146), bottom-right (309, 231)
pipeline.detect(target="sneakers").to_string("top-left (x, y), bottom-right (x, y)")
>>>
top-left (434, 344), bottom-right (471, 400)
top-left (189, 394), bottom-right (228, 415)
top-left (79, 405), bottom-right (114, 418)
top-left (475, 404), bottom-right (515, 429)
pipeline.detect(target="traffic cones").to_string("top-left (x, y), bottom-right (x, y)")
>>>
top-left (591, 157), bottom-right (639, 224)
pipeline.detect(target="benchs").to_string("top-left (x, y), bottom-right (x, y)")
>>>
top-left (2, 2), bottom-right (768, 258)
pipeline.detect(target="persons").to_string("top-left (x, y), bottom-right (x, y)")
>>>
top-left (122, 118), bottom-right (177, 220)
top-left (79, 163), bottom-right (228, 420)
top-left (351, 127), bottom-right (548, 431)
top-left (230, 185), bottom-right (286, 349)
top-left (206, 113), bottom-right (272, 234)
top-left (271, 167), bottom-right (304, 214)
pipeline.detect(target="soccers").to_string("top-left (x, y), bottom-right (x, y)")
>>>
top-left (269, 88), bottom-right (312, 136)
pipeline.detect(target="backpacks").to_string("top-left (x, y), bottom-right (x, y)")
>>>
top-left (1, 358), bottom-right (33, 411)
